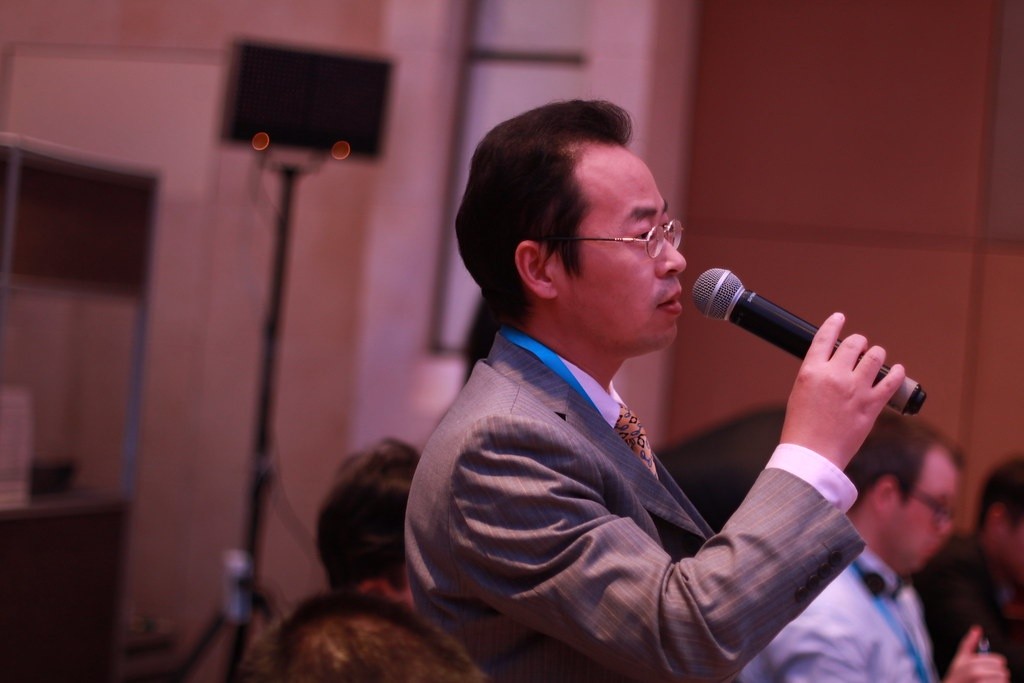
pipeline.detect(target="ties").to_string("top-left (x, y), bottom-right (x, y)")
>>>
top-left (616, 404), bottom-right (659, 479)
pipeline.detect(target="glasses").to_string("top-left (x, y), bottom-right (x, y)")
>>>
top-left (527, 219), bottom-right (684, 259)
top-left (898, 482), bottom-right (955, 531)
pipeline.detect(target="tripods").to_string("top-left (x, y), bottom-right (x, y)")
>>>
top-left (174, 159), bottom-right (306, 683)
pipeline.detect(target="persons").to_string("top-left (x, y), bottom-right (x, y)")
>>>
top-left (404, 99), bottom-right (906, 683)
top-left (232, 407), bottom-right (1024, 683)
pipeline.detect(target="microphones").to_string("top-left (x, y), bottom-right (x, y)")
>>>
top-left (693, 267), bottom-right (926, 416)
